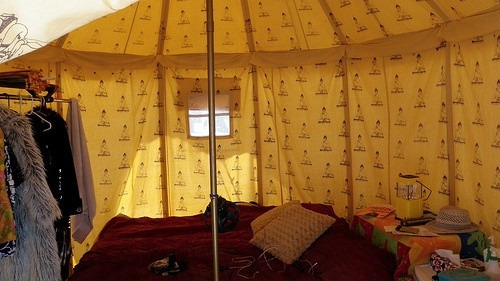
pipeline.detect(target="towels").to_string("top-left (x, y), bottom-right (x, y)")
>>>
top-left (66, 97), bottom-right (97, 244)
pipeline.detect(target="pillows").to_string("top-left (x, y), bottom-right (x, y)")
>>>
top-left (248, 204), bottom-right (336, 265)
top-left (251, 199), bottom-right (300, 235)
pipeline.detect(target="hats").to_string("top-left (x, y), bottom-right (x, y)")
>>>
top-left (424, 204), bottom-right (480, 233)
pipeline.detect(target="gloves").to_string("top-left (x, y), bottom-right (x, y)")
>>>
top-left (147, 252), bottom-right (188, 277)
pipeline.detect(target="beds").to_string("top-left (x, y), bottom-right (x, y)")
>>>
top-left (70, 201), bottom-right (401, 281)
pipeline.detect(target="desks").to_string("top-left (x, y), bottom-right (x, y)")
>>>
top-left (412, 258), bottom-right (500, 281)
top-left (352, 205), bottom-right (490, 281)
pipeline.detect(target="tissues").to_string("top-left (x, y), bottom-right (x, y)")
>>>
top-left (430, 248), bottom-right (464, 273)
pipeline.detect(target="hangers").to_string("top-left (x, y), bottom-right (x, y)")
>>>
top-left (0, 91), bottom-right (68, 137)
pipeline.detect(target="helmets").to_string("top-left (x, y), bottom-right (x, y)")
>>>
top-left (204, 197), bottom-right (238, 231)
